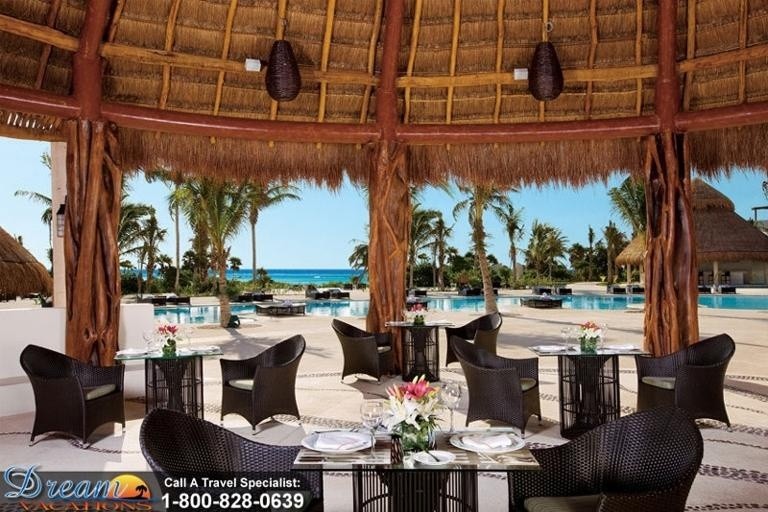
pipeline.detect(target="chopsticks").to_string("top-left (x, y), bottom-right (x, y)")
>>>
top-left (299, 457), bottom-right (360, 462)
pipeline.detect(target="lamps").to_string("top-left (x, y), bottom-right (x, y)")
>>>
top-left (526, 43), bottom-right (564, 100)
top-left (263, 39), bottom-right (301, 102)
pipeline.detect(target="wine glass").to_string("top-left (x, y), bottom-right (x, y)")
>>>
top-left (561, 326), bottom-right (573, 351)
top-left (184, 326), bottom-right (195, 351)
top-left (597, 323), bottom-right (607, 349)
top-left (143, 329), bottom-right (156, 353)
top-left (443, 384), bottom-right (461, 435)
top-left (361, 401), bottom-right (383, 461)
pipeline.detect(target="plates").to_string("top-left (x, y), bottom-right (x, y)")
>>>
top-left (301, 430), bottom-right (376, 454)
top-left (532, 344), bottom-right (565, 352)
top-left (449, 430), bottom-right (525, 454)
top-left (413, 449), bottom-right (457, 465)
top-left (189, 346), bottom-right (220, 352)
top-left (116, 348), bottom-right (148, 356)
top-left (606, 344), bottom-right (640, 350)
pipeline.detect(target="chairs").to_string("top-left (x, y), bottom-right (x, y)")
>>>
top-left (636, 333), bottom-right (737, 433)
top-left (509, 407), bottom-right (704, 511)
top-left (446, 312), bottom-right (502, 366)
top-left (219, 335), bottom-right (308, 436)
top-left (140, 407), bottom-right (326, 512)
top-left (331, 317), bottom-right (398, 386)
top-left (19, 343), bottom-right (127, 449)
top-left (455, 338), bottom-right (544, 440)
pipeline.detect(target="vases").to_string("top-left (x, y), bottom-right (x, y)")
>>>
top-left (414, 317), bottom-right (424, 323)
top-left (161, 343), bottom-right (180, 356)
top-left (400, 418), bottom-right (439, 456)
top-left (580, 334), bottom-right (598, 353)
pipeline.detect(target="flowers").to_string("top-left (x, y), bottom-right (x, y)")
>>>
top-left (385, 374), bottom-right (445, 431)
top-left (159, 321), bottom-right (178, 340)
top-left (411, 304), bottom-right (425, 314)
top-left (578, 320), bottom-right (604, 339)
